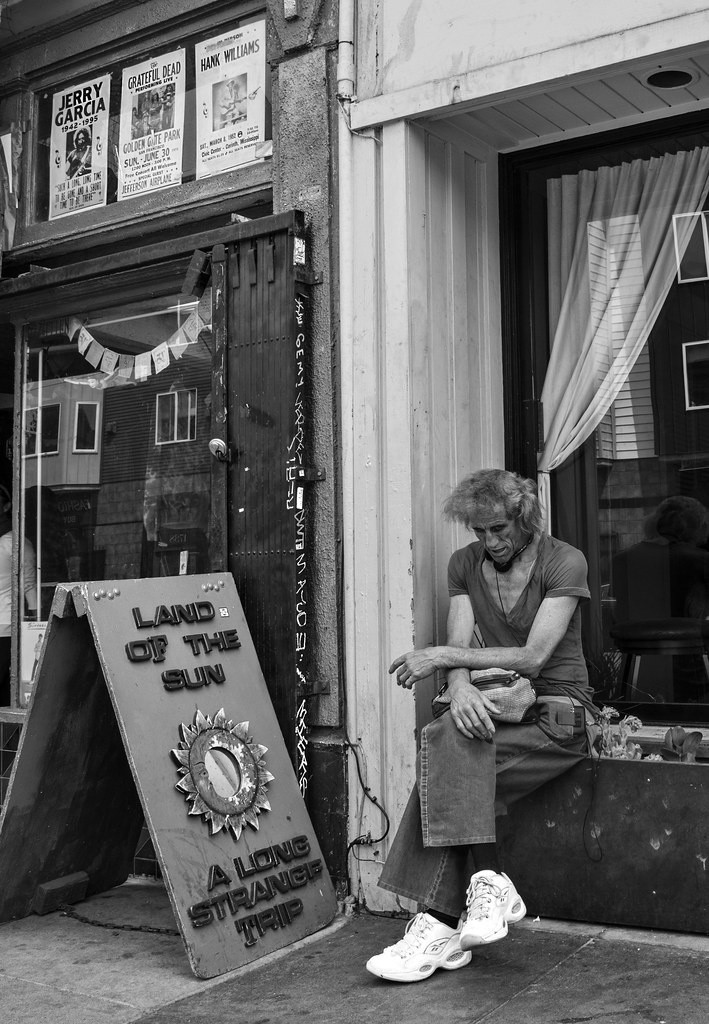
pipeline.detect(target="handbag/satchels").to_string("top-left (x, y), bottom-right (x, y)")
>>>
top-left (432, 668), bottom-right (540, 723)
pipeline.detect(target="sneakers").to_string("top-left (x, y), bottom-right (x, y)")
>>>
top-left (460, 870), bottom-right (525, 951)
top-left (366, 912), bottom-right (472, 981)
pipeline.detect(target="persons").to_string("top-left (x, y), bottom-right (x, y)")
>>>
top-left (0, 487), bottom-right (54, 708)
top-left (608, 496), bottom-right (709, 653)
top-left (367, 467), bottom-right (598, 982)
